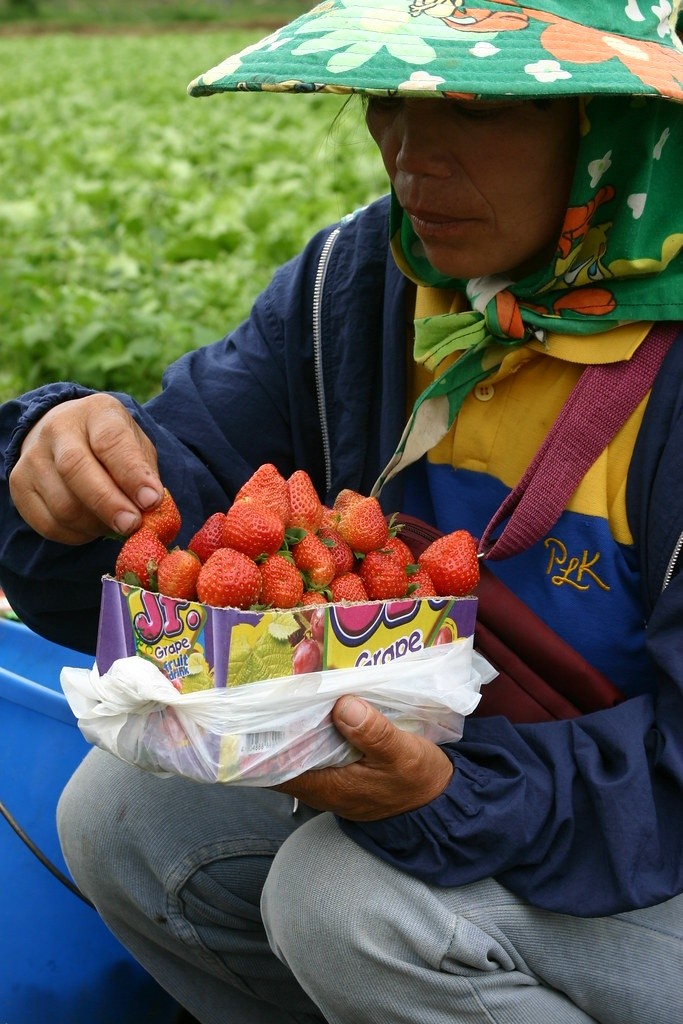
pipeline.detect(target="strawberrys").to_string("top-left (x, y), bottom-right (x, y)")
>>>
top-left (114, 464), bottom-right (480, 610)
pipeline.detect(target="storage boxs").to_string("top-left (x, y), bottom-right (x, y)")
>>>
top-left (78, 575), bottom-right (478, 784)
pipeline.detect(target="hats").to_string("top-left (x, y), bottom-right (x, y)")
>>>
top-left (187, 0), bottom-right (682, 103)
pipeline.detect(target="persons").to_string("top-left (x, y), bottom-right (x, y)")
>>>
top-left (0, 1), bottom-right (682, 1024)
